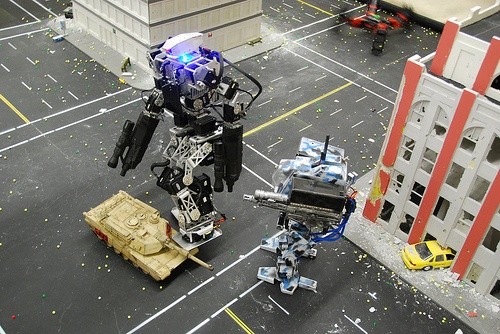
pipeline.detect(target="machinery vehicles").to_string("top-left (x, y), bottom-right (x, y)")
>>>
top-left (83, 190), bottom-right (214, 282)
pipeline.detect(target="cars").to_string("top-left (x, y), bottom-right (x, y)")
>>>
top-left (401, 239), bottom-right (457, 270)
